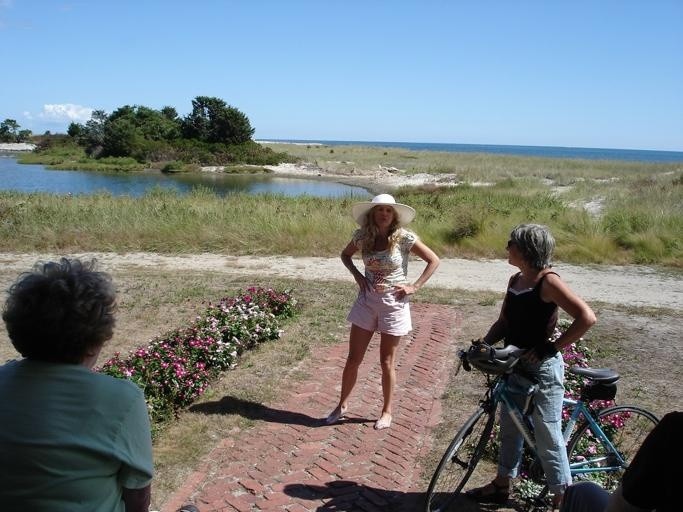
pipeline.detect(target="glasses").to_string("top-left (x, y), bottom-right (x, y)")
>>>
top-left (506, 239), bottom-right (517, 248)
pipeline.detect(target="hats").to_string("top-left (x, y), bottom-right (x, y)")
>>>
top-left (349, 193), bottom-right (417, 228)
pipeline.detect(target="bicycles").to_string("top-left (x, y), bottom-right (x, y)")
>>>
top-left (424, 337), bottom-right (662, 512)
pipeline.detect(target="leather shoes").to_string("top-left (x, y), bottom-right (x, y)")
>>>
top-left (324, 404), bottom-right (347, 426)
top-left (374, 415), bottom-right (392, 430)
top-left (466, 480), bottom-right (513, 503)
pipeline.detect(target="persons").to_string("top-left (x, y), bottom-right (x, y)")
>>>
top-left (0, 257), bottom-right (152, 512)
top-left (323, 192), bottom-right (440, 430)
top-left (461, 220), bottom-right (596, 512)
top-left (557, 409), bottom-right (683, 512)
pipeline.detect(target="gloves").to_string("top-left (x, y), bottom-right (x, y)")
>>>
top-left (534, 341), bottom-right (558, 362)
